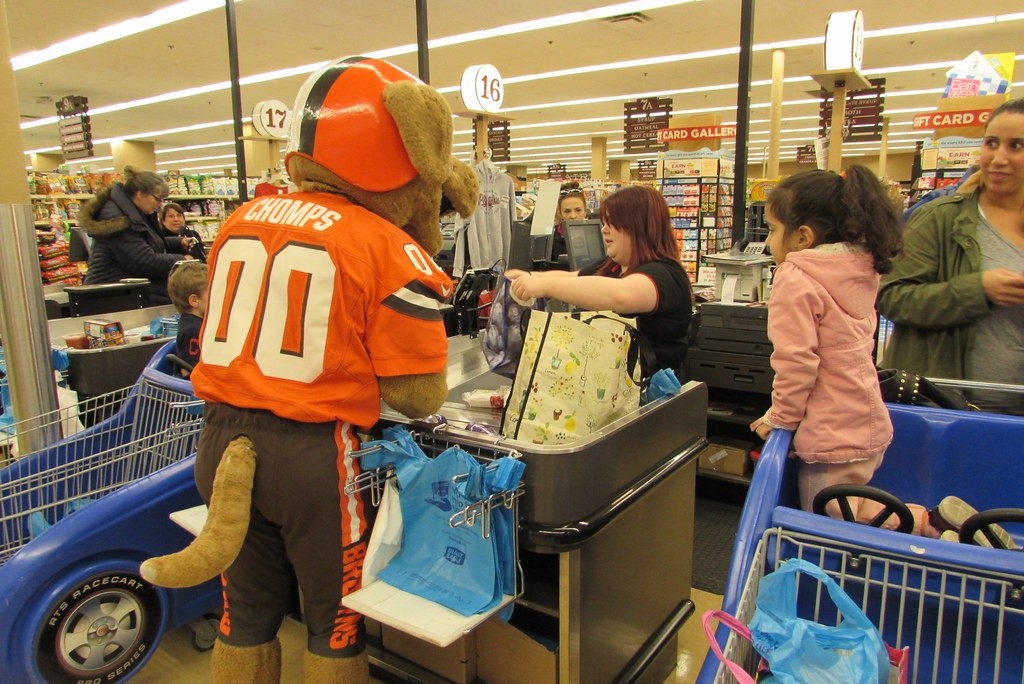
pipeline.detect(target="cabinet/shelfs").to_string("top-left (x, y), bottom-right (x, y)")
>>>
top-left (31, 193), bottom-right (255, 244)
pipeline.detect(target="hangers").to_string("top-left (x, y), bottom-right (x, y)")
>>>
top-left (484, 147), bottom-right (494, 171)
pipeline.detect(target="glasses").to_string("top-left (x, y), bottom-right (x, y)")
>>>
top-left (145, 189), bottom-right (164, 204)
top-left (560, 189), bottom-right (582, 195)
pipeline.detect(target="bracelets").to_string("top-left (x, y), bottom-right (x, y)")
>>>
top-left (528, 271), bottom-right (532, 276)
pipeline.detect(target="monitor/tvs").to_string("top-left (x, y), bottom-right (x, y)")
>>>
top-left (69, 227), bottom-right (93, 263)
top-left (563, 219), bottom-right (608, 272)
top-left (506, 220), bottom-right (555, 271)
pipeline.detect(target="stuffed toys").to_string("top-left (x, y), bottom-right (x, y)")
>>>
top-left (139, 55), bottom-right (480, 684)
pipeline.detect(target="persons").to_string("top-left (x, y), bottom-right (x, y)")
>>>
top-left (750, 163), bottom-right (1015, 549)
top-left (76, 166), bottom-right (195, 308)
top-left (159, 203), bottom-right (207, 265)
top-left (504, 186), bottom-right (692, 386)
top-left (874, 97), bottom-right (1024, 416)
top-left (902, 165), bottom-right (980, 223)
top-left (550, 181), bottom-right (587, 263)
top-left (167, 262), bottom-right (207, 381)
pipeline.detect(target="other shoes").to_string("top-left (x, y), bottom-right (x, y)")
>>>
top-left (940, 530), bottom-right (961, 543)
top-left (932, 495), bottom-right (1016, 551)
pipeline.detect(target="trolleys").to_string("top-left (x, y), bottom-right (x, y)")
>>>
top-left (696, 403), bottom-right (1023, 684)
top-left (1, 339), bottom-right (221, 684)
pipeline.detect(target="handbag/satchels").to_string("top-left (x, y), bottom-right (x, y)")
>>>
top-left (497, 308), bottom-right (657, 445)
top-left (876, 368), bottom-right (983, 413)
top-left (453, 259), bottom-right (507, 337)
top-left (481, 273), bottom-right (541, 379)
top-left (701, 557), bottom-right (911, 684)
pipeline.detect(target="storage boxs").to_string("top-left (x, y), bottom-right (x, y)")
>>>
top-left (86, 334), bottom-right (126, 349)
top-left (83, 318), bottom-right (125, 341)
